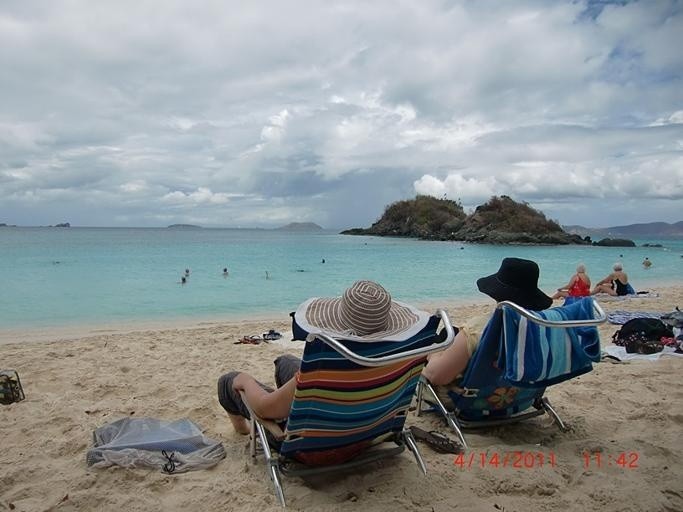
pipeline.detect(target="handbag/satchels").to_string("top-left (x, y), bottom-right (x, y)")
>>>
top-left (617, 317), bottom-right (674, 341)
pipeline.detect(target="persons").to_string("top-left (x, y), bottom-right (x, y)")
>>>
top-left (180, 275), bottom-right (187, 283)
top-left (217, 280), bottom-right (430, 435)
top-left (592, 262), bottom-right (628, 297)
top-left (642, 257), bottom-right (652, 267)
top-left (418, 257), bottom-right (553, 410)
top-left (223, 267), bottom-right (228, 275)
top-left (184, 268), bottom-right (189, 276)
top-left (550, 262), bottom-right (591, 300)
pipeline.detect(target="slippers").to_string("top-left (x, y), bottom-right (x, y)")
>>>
top-left (409, 424), bottom-right (467, 455)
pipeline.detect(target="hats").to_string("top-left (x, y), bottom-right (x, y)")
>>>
top-left (476, 259), bottom-right (553, 312)
top-left (295, 279), bottom-right (433, 345)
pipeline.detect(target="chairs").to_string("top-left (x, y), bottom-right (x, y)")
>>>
top-left (240, 309), bottom-right (467, 509)
top-left (414, 297), bottom-right (607, 450)
